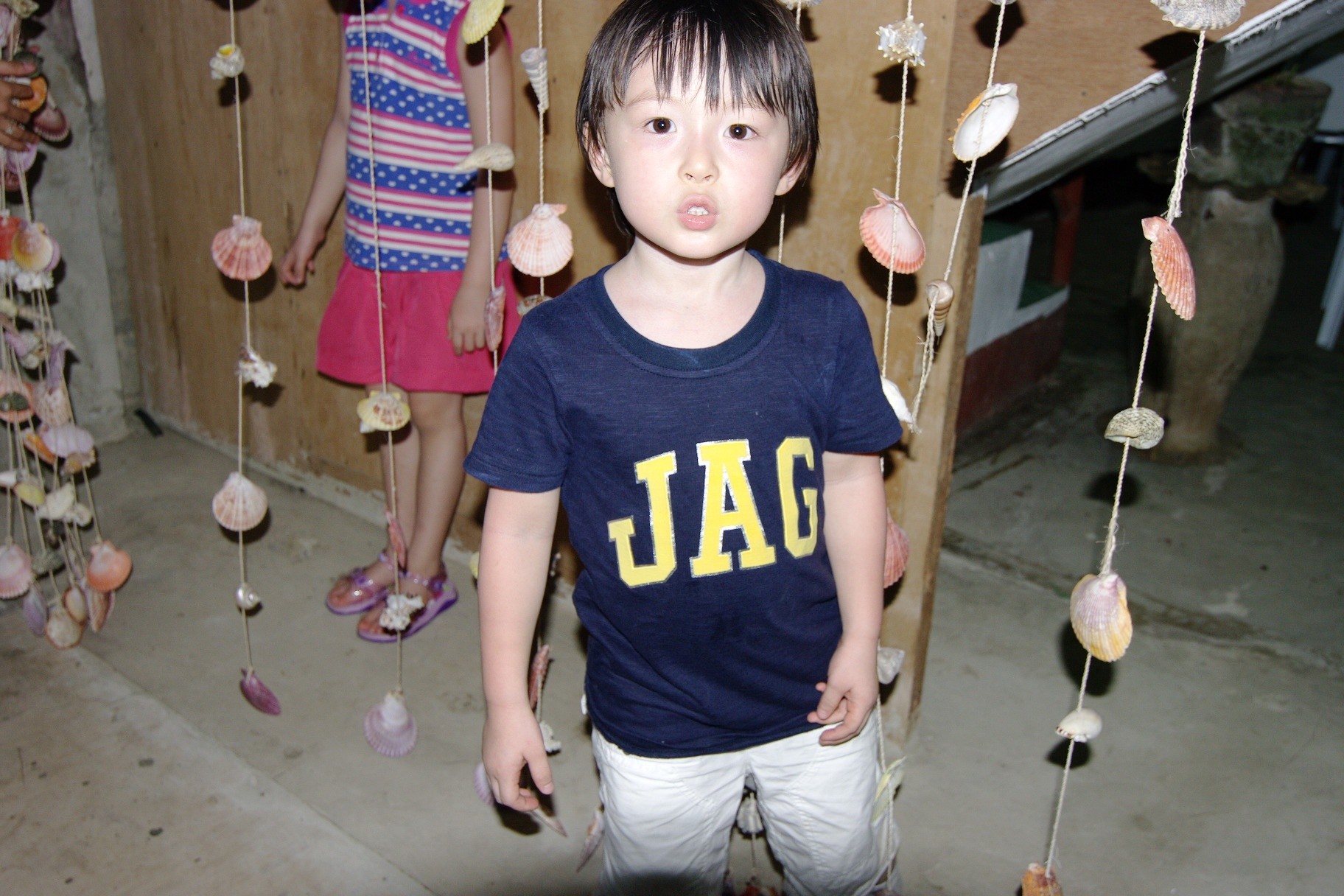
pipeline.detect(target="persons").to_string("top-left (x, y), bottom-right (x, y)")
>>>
top-left (462, 1), bottom-right (903, 896)
top-left (281, 0), bottom-right (511, 642)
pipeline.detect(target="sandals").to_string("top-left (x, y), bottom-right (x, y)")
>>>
top-left (357, 563), bottom-right (458, 643)
top-left (325, 549), bottom-right (405, 615)
